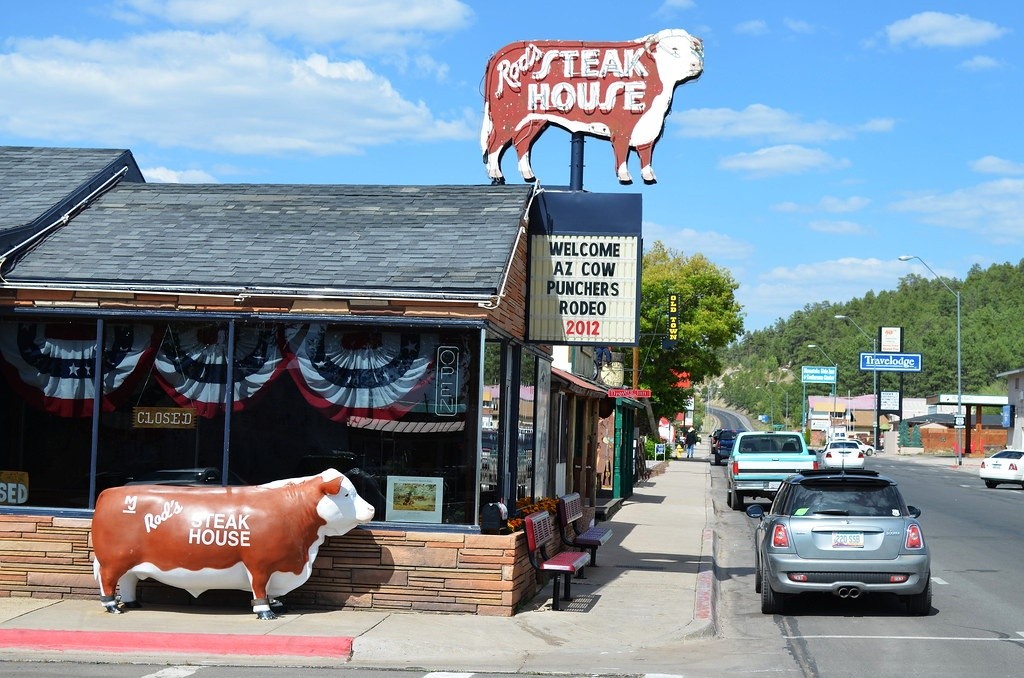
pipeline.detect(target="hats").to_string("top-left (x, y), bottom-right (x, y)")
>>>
top-left (688, 427), bottom-right (695, 433)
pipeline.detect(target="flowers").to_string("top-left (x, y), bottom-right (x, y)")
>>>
top-left (506, 494), bottom-right (560, 533)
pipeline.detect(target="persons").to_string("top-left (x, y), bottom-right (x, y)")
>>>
top-left (594, 346), bottom-right (612, 369)
top-left (686, 427), bottom-right (696, 458)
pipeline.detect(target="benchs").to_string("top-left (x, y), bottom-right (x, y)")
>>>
top-left (523, 511), bottom-right (591, 611)
top-left (556, 492), bottom-right (614, 566)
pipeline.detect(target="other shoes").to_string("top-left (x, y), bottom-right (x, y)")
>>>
top-left (687, 454), bottom-right (689, 458)
top-left (691, 455), bottom-right (693, 457)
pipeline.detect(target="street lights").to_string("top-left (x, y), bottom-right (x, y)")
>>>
top-left (835, 313), bottom-right (876, 451)
top-left (770, 381), bottom-right (788, 431)
top-left (707, 377), bottom-right (719, 431)
top-left (705, 392), bottom-right (721, 435)
top-left (807, 345), bottom-right (837, 442)
top-left (781, 369), bottom-right (806, 438)
top-left (898, 255), bottom-right (962, 466)
top-left (756, 387), bottom-right (773, 431)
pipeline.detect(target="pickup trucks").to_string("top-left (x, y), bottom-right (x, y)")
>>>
top-left (482, 428), bottom-right (528, 499)
top-left (715, 431), bottom-right (745, 464)
top-left (724, 431), bottom-right (819, 510)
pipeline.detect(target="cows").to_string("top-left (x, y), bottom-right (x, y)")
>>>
top-left (479, 27), bottom-right (706, 186)
top-left (90, 467), bottom-right (376, 621)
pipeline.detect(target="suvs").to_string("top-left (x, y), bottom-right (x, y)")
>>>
top-left (832, 438), bottom-right (876, 457)
top-left (709, 429), bottom-right (727, 452)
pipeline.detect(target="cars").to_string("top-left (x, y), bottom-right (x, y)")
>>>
top-left (302, 436), bottom-right (410, 473)
top-left (978, 450), bottom-right (1024, 491)
top-left (746, 465), bottom-right (933, 614)
top-left (125, 466), bottom-right (225, 485)
top-left (819, 441), bottom-right (864, 470)
